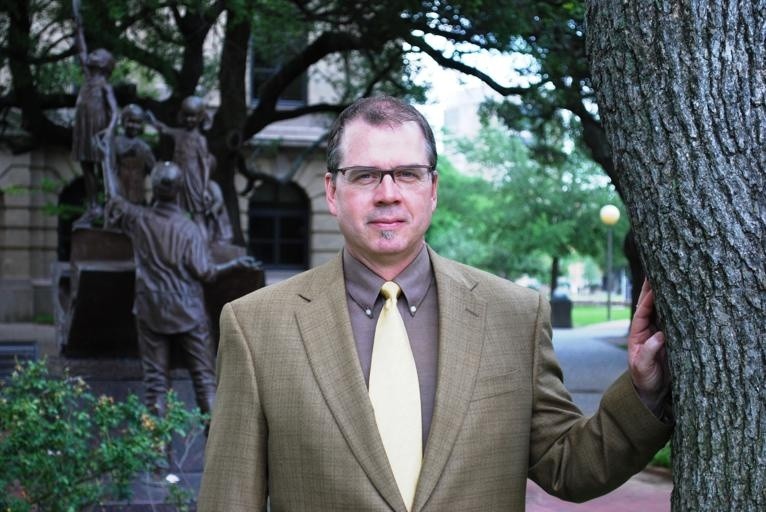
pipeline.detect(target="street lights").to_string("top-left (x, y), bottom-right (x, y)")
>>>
top-left (600, 204), bottom-right (621, 322)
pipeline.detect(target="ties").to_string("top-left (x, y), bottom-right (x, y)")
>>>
top-left (368, 282), bottom-right (424, 512)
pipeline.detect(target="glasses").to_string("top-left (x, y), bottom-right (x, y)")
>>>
top-left (329, 163), bottom-right (436, 191)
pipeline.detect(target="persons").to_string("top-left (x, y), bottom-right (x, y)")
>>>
top-left (204, 151), bottom-right (234, 245)
top-left (72, 13), bottom-right (120, 227)
top-left (115, 102), bottom-right (155, 207)
top-left (196, 94), bottom-right (675, 511)
top-left (91, 133), bottom-right (265, 475)
top-left (147, 95), bottom-right (211, 242)
